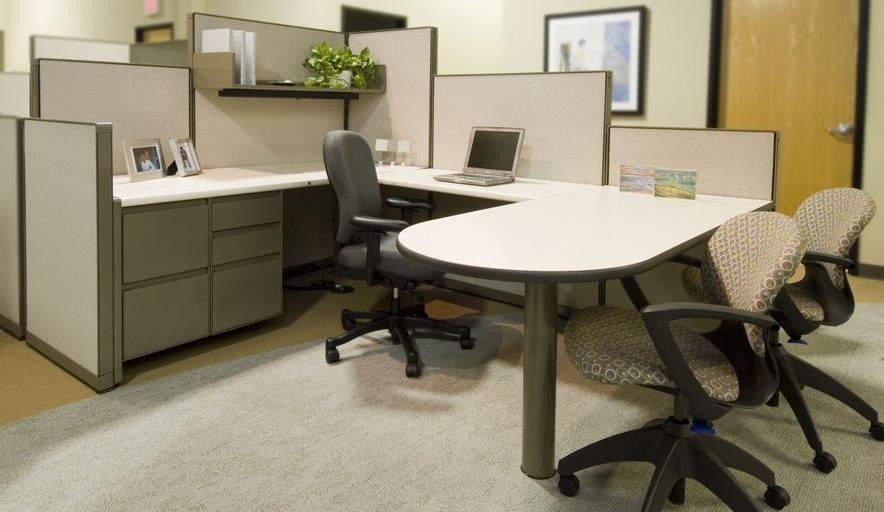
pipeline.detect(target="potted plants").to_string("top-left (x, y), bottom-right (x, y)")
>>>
top-left (302, 39), bottom-right (377, 90)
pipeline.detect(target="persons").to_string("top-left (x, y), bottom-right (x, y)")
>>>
top-left (138, 150), bottom-right (155, 170)
top-left (180, 146), bottom-right (193, 168)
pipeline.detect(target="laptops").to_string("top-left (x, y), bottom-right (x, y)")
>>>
top-left (433, 125), bottom-right (525, 186)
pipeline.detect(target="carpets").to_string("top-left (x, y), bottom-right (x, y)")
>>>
top-left (2, 298), bottom-right (882, 512)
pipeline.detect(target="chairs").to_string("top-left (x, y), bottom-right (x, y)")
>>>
top-left (556, 186), bottom-right (884, 510)
top-left (319, 130), bottom-right (474, 382)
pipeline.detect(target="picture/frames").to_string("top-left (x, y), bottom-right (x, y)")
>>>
top-left (120, 137), bottom-right (202, 185)
top-left (543, 5), bottom-right (648, 116)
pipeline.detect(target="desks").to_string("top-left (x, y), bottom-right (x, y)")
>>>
top-left (557, 185), bottom-right (770, 286)
top-left (114, 159), bottom-right (776, 480)
